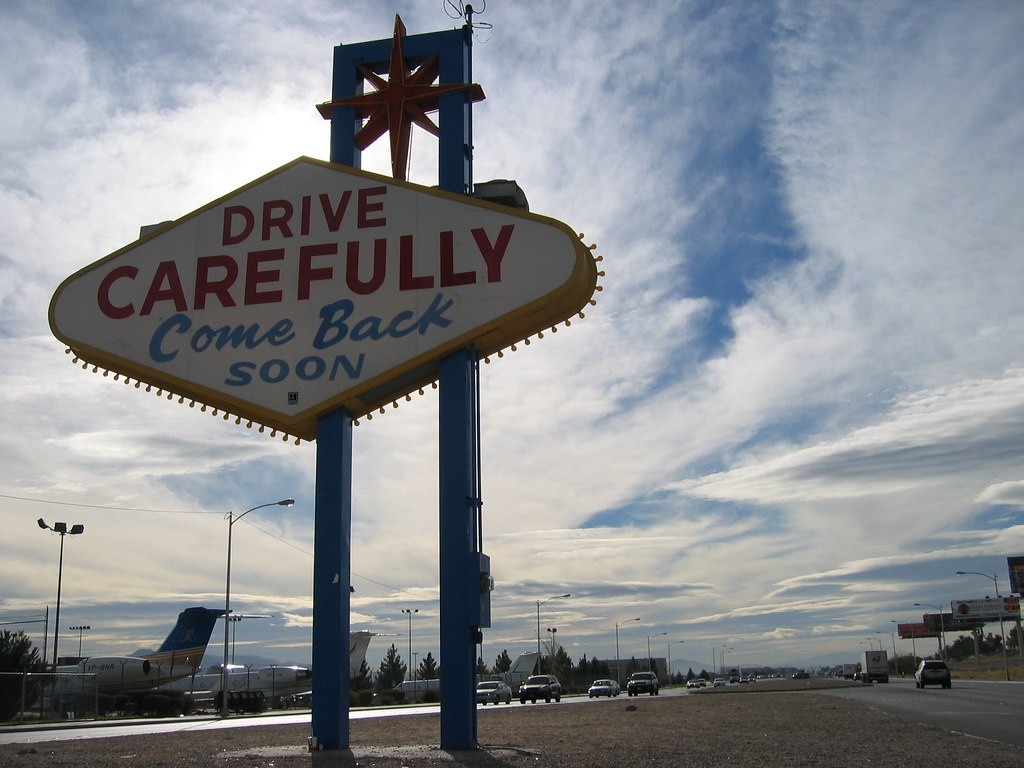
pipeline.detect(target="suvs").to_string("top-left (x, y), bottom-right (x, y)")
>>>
top-left (518, 673), bottom-right (561, 704)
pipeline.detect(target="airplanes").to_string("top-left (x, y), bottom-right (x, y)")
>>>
top-left (45, 606), bottom-right (309, 715)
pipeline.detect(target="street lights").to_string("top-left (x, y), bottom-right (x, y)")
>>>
top-left (860, 620), bottom-right (917, 668)
top-left (956, 571), bottom-right (1009, 681)
top-left (37, 518), bottom-right (84, 721)
top-left (69, 626), bottom-right (90, 664)
top-left (402, 609), bottom-right (418, 681)
top-left (537, 594), bottom-right (571, 675)
top-left (647, 632), bottom-right (684, 688)
top-left (221, 498), bottom-right (295, 717)
top-left (229, 618), bottom-right (242, 673)
top-left (616, 617), bottom-right (640, 684)
top-left (913, 603), bottom-right (948, 663)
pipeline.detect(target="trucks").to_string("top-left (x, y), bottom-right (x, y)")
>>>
top-left (838, 651), bottom-right (889, 683)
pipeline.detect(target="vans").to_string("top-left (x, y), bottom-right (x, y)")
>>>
top-left (393, 679), bottom-right (440, 696)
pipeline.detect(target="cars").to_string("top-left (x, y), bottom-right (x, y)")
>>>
top-left (476, 681), bottom-right (512, 705)
top-left (627, 672), bottom-right (659, 697)
top-left (686, 667), bottom-right (809, 688)
top-left (588, 679), bottom-right (620, 698)
top-left (915, 660), bottom-right (951, 689)
